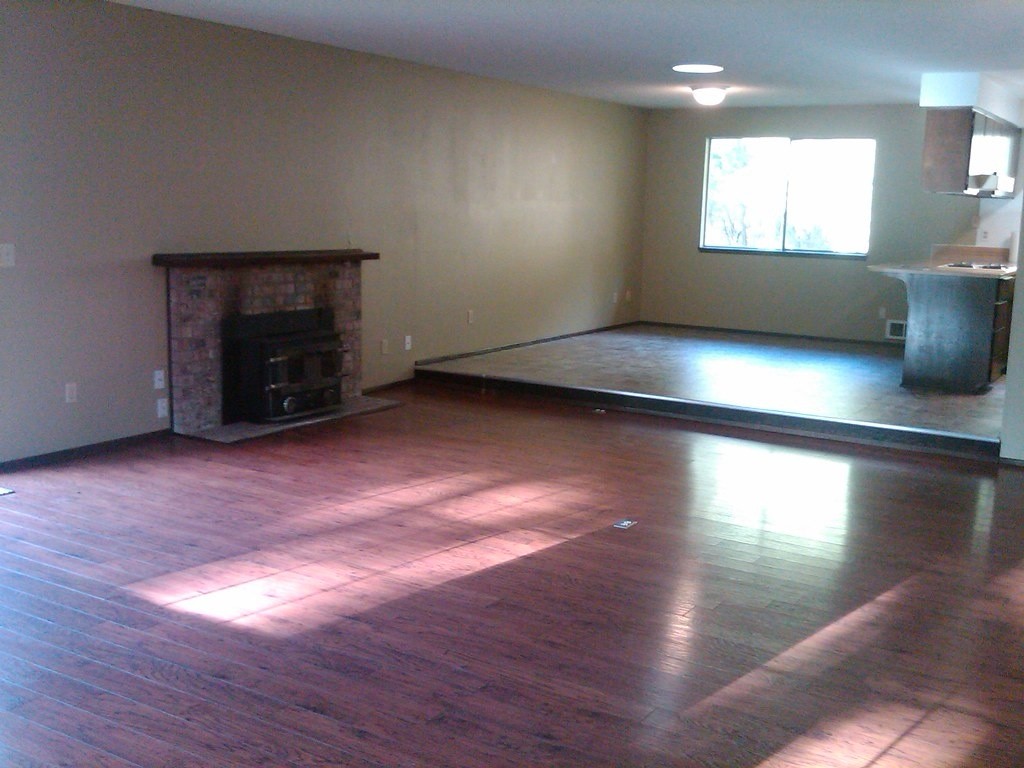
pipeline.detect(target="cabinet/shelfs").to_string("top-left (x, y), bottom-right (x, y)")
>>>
top-left (918, 106), bottom-right (1021, 200)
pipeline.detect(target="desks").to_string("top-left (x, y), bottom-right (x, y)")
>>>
top-left (865, 262), bottom-right (1016, 396)
